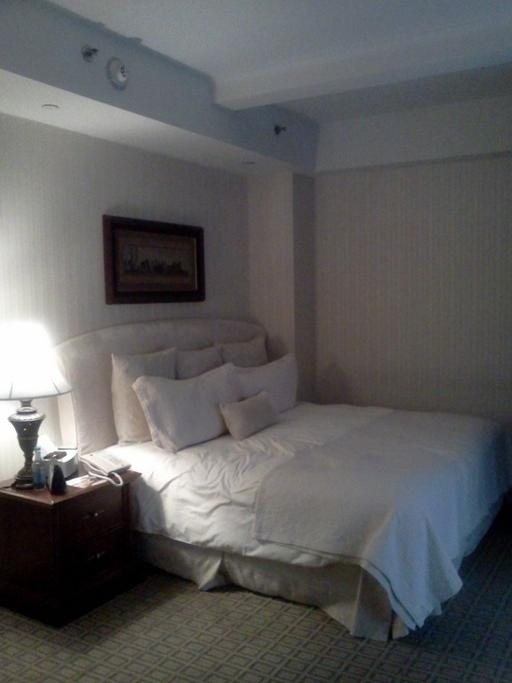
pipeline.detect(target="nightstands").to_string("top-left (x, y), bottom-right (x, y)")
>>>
top-left (1, 469), bottom-right (144, 631)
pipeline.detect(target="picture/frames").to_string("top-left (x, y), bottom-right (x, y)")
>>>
top-left (101, 214), bottom-right (208, 304)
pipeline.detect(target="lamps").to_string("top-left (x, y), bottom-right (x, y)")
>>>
top-left (0, 321), bottom-right (74, 490)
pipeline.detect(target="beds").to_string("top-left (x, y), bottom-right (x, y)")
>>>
top-left (48, 316), bottom-right (502, 618)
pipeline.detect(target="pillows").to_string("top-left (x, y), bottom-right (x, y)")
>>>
top-left (110, 333), bottom-right (299, 455)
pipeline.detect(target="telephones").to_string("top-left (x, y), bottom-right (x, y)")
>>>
top-left (79, 450), bottom-right (132, 477)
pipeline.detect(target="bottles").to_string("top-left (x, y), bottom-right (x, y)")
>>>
top-left (32, 446), bottom-right (47, 491)
